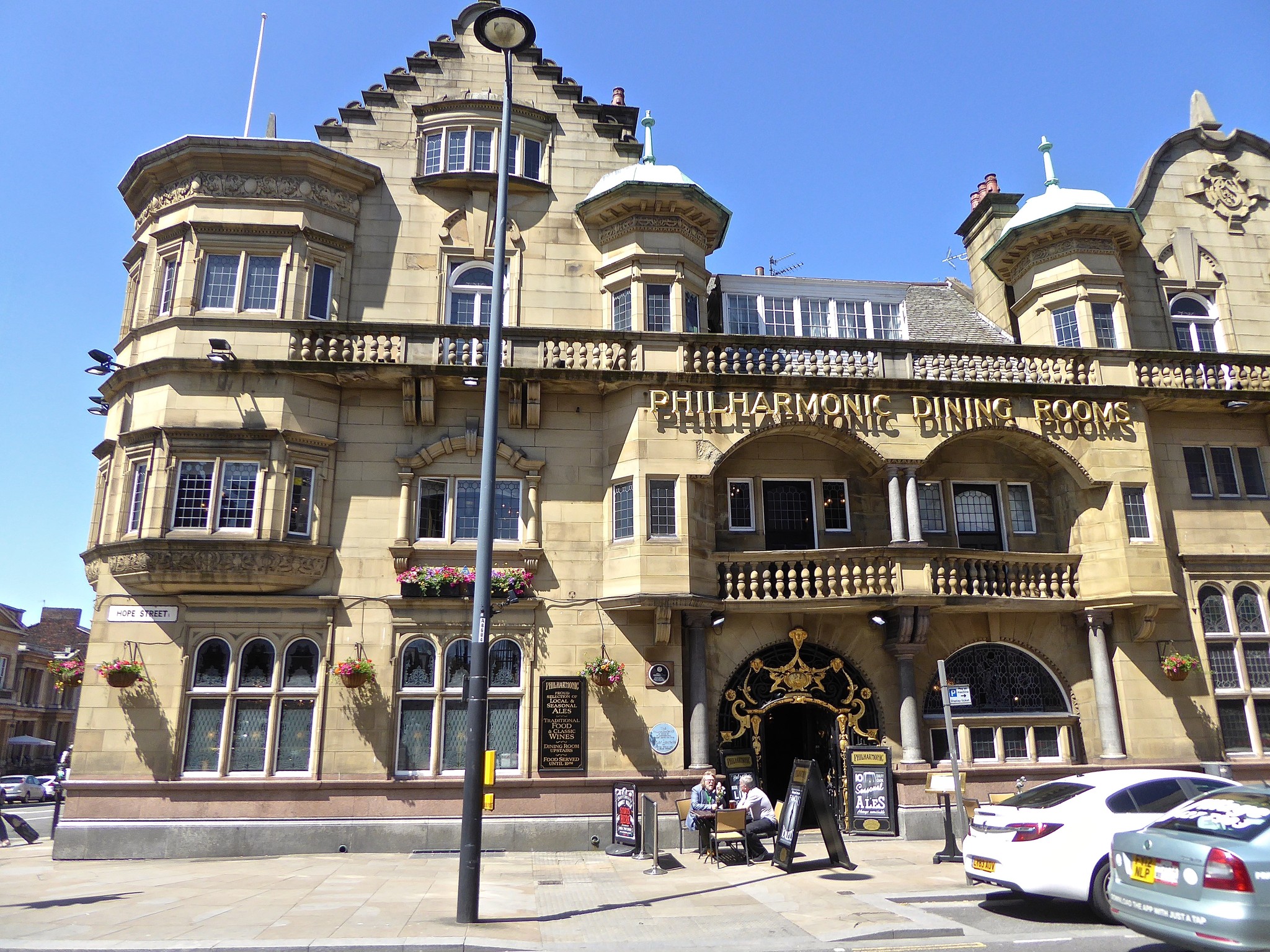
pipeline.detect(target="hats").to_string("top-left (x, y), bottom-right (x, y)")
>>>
top-left (68, 744), bottom-right (73, 749)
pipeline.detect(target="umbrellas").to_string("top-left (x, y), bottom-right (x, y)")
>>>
top-left (8, 735), bottom-right (56, 767)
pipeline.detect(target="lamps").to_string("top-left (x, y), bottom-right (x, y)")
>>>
top-left (84, 349), bottom-right (125, 375)
top-left (87, 396), bottom-right (109, 416)
top-left (867, 610), bottom-right (887, 631)
top-left (206, 338), bottom-right (237, 363)
top-left (1222, 400), bottom-right (1249, 408)
top-left (711, 614), bottom-right (725, 635)
top-left (462, 376), bottom-right (480, 387)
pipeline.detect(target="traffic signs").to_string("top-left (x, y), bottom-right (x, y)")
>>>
top-left (947, 687), bottom-right (972, 706)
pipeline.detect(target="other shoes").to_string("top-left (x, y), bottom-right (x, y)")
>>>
top-left (753, 850), bottom-right (769, 861)
top-left (0, 842), bottom-right (11, 847)
top-left (707, 848), bottom-right (721, 857)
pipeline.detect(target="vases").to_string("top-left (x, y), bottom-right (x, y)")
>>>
top-left (400, 581), bottom-right (525, 598)
top-left (340, 673), bottom-right (367, 688)
top-left (1164, 667), bottom-right (1189, 681)
top-left (59, 673), bottom-right (82, 686)
top-left (717, 801), bottom-right (721, 809)
top-left (106, 671), bottom-right (136, 687)
top-left (591, 671), bottom-right (614, 685)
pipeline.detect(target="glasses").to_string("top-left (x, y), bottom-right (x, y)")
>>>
top-left (704, 779), bottom-right (715, 782)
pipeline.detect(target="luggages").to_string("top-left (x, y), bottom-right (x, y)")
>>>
top-left (0, 813), bottom-right (39, 844)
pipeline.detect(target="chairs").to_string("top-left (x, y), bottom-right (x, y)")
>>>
top-left (674, 797), bottom-right (784, 869)
top-left (962, 792), bottom-right (1016, 824)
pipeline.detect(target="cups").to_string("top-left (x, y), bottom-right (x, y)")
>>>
top-left (729, 800), bottom-right (737, 809)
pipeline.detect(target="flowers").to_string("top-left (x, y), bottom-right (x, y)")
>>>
top-left (326, 657), bottom-right (377, 684)
top-left (576, 657), bottom-right (628, 683)
top-left (712, 782), bottom-right (726, 803)
top-left (1160, 651), bottom-right (1201, 674)
top-left (396, 562), bottom-right (533, 596)
top-left (93, 658), bottom-right (155, 688)
top-left (47, 657), bottom-right (86, 694)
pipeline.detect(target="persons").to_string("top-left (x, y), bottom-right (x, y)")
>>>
top-left (686, 771), bottom-right (726, 857)
top-left (736, 775), bottom-right (776, 862)
top-left (0, 786), bottom-right (11, 847)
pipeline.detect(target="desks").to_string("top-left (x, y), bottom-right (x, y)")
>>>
top-left (689, 809), bottom-right (749, 864)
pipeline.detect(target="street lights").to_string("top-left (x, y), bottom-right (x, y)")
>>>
top-left (452, 8), bottom-right (538, 922)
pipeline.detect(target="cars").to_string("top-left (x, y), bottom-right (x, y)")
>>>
top-left (1106, 773), bottom-right (1270, 952)
top-left (36, 775), bottom-right (64, 802)
top-left (962, 766), bottom-right (1243, 923)
top-left (0, 775), bottom-right (46, 804)
